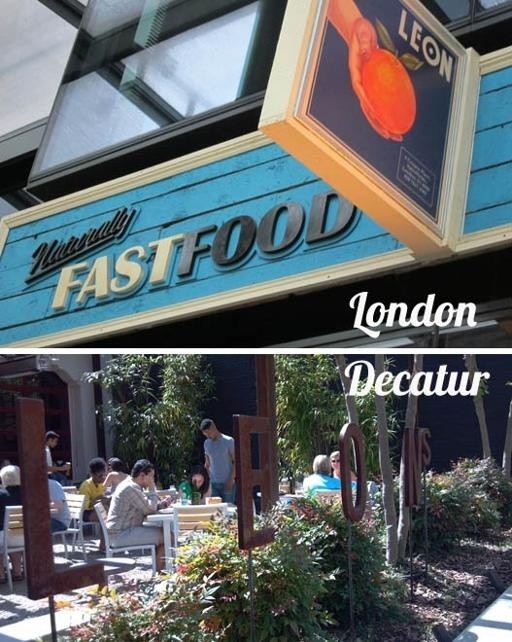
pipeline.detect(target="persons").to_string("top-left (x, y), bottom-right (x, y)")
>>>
top-left (48, 478), bottom-right (71, 533)
top-left (330, 451), bottom-right (357, 480)
top-left (201, 418), bottom-right (238, 504)
top-left (302, 454), bottom-right (343, 495)
top-left (106, 459), bottom-right (173, 575)
top-left (103, 458), bottom-right (129, 496)
top-left (0, 459), bottom-right (25, 585)
top-left (46, 432), bottom-right (71, 486)
top-left (106, 457), bottom-right (118, 478)
top-left (80, 456), bottom-right (113, 554)
top-left (186, 465), bottom-right (212, 505)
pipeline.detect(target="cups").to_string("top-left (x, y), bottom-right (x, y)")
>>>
top-left (66, 462), bottom-right (71, 476)
top-left (192, 492), bottom-right (201, 505)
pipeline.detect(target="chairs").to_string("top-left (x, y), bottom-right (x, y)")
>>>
top-left (1, 487), bottom-right (227, 594)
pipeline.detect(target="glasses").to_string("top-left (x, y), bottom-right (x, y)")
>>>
top-left (332, 460), bottom-right (340, 462)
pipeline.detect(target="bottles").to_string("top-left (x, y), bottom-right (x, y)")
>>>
top-left (181, 486), bottom-right (188, 506)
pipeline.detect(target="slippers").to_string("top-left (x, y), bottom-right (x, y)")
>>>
top-left (0, 574), bottom-right (24, 584)
